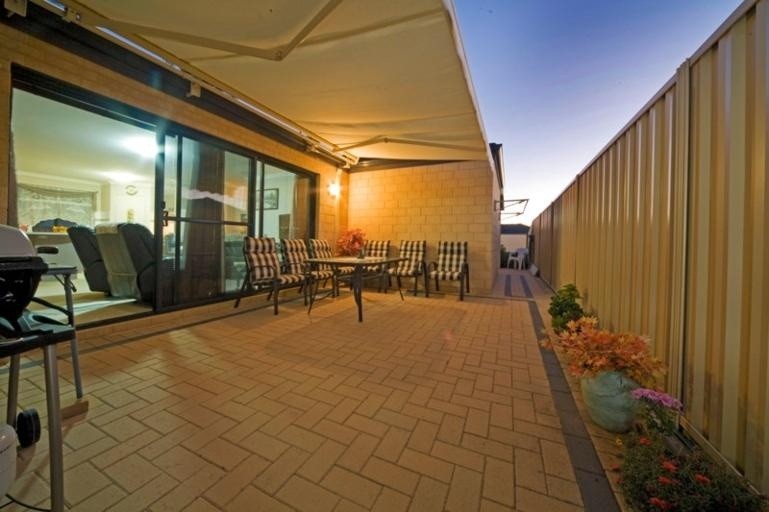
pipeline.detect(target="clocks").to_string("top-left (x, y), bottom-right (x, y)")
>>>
top-left (125, 185), bottom-right (138, 195)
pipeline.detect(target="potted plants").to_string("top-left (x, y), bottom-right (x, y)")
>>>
top-left (539, 316), bottom-right (668, 434)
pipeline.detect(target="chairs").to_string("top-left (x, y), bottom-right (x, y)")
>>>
top-left (365, 239), bottom-right (392, 293)
top-left (507, 247), bottom-right (529, 270)
top-left (234, 238), bottom-right (307, 315)
top-left (66, 225), bottom-right (110, 296)
top-left (298, 238), bottom-right (354, 293)
top-left (95, 223), bottom-right (180, 304)
top-left (385, 240), bottom-right (426, 297)
top-left (425, 240), bottom-right (469, 300)
top-left (266, 239), bottom-right (335, 301)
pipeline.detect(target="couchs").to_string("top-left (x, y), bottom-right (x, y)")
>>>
top-left (222, 240), bottom-right (246, 280)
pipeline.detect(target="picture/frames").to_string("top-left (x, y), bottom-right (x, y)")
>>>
top-left (255, 188), bottom-right (279, 210)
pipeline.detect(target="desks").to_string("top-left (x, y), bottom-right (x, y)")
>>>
top-left (1, 310), bottom-right (75, 512)
top-left (303, 255), bottom-right (409, 322)
top-left (42, 264), bottom-right (83, 399)
